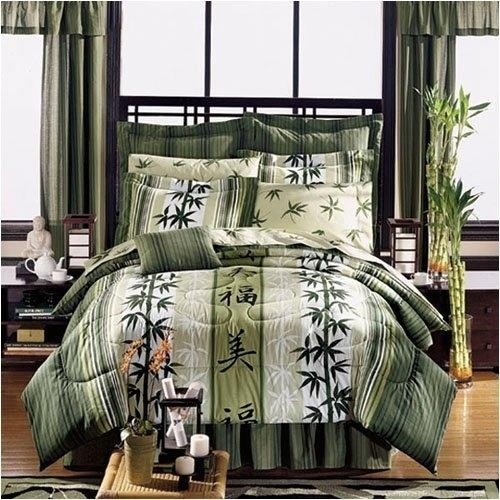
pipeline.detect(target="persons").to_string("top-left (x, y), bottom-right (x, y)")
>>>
top-left (23, 215), bottom-right (53, 259)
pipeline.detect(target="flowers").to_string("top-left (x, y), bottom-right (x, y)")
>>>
top-left (120, 336), bottom-right (171, 442)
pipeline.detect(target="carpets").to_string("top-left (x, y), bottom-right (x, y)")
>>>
top-left (0, 475), bottom-right (499, 499)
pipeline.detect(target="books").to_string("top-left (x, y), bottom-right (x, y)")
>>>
top-left (14, 307), bottom-right (50, 313)
top-left (6, 347), bottom-right (60, 352)
top-left (5, 342), bottom-right (60, 348)
top-left (18, 313), bottom-right (52, 317)
top-left (4, 351), bottom-right (53, 357)
top-left (17, 316), bottom-right (56, 320)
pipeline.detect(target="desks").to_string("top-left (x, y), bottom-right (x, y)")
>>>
top-left (96, 448), bottom-right (230, 499)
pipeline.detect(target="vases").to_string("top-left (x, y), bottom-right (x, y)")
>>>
top-left (123, 428), bottom-right (158, 485)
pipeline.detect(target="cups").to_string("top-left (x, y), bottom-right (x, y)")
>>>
top-left (410, 272), bottom-right (428, 284)
top-left (50, 268), bottom-right (68, 281)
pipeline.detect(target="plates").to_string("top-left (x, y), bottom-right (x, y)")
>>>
top-left (46, 276), bottom-right (74, 284)
top-left (408, 278), bottom-right (433, 287)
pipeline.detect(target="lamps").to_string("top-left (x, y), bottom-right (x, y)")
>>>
top-left (386, 218), bottom-right (421, 280)
top-left (63, 214), bottom-right (98, 277)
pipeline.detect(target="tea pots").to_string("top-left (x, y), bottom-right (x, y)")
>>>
top-left (24, 252), bottom-right (66, 279)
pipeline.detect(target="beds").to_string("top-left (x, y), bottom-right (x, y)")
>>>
top-left (20, 92), bottom-right (459, 478)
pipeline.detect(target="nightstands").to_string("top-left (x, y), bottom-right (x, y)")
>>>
top-left (412, 270), bottom-right (499, 372)
top-left (1, 258), bottom-right (88, 370)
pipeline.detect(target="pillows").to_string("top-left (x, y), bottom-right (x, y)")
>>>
top-left (132, 224), bottom-right (222, 274)
top-left (238, 111), bottom-right (385, 247)
top-left (127, 152), bottom-right (264, 178)
top-left (117, 116), bottom-right (240, 176)
top-left (236, 149), bottom-right (374, 227)
top-left (113, 172), bottom-right (259, 246)
top-left (251, 181), bottom-right (374, 255)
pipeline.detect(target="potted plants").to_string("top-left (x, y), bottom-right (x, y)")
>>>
top-left (419, 173), bottom-right (485, 389)
top-left (411, 83), bottom-right (491, 282)
